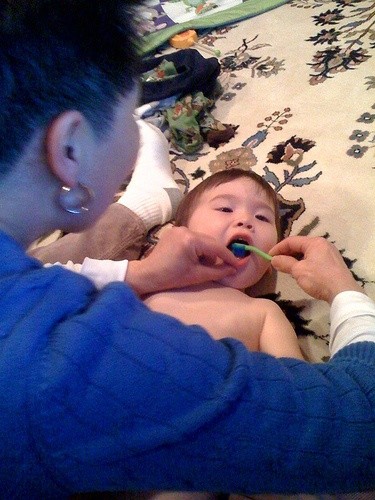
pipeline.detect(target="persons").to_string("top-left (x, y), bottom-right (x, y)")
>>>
top-left (0, 0), bottom-right (375, 500)
top-left (141, 168), bottom-right (310, 500)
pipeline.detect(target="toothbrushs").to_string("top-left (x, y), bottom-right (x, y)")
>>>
top-left (232, 243), bottom-right (272, 263)
